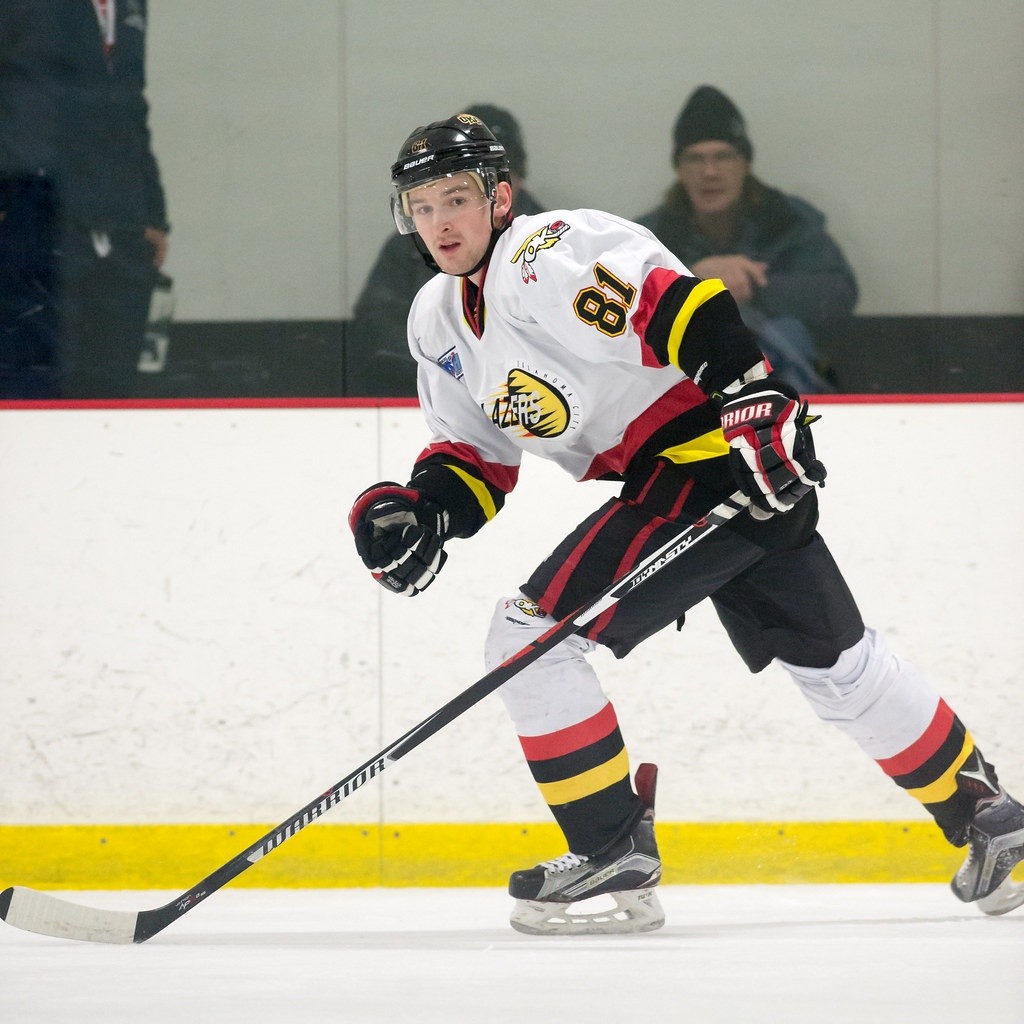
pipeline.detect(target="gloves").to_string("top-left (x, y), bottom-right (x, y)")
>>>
top-left (348, 480), bottom-right (451, 598)
top-left (720, 379), bottom-right (824, 515)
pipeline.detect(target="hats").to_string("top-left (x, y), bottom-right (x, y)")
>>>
top-left (673, 86), bottom-right (752, 168)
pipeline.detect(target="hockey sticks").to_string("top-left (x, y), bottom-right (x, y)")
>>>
top-left (0, 487), bottom-right (754, 945)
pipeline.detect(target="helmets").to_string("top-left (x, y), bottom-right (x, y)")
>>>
top-left (390, 113), bottom-right (511, 236)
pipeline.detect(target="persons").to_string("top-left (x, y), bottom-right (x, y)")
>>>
top-left (637, 84), bottom-right (858, 394)
top-left (0, 0), bottom-right (171, 400)
top-left (339, 103), bottom-right (548, 398)
top-left (347, 115), bottom-right (1024, 936)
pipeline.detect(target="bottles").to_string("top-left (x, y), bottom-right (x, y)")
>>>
top-left (138, 276), bottom-right (174, 373)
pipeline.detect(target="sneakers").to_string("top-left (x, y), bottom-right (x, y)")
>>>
top-left (950, 745), bottom-right (1024, 916)
top-left (508, 763), bottom-right (666, 935)
